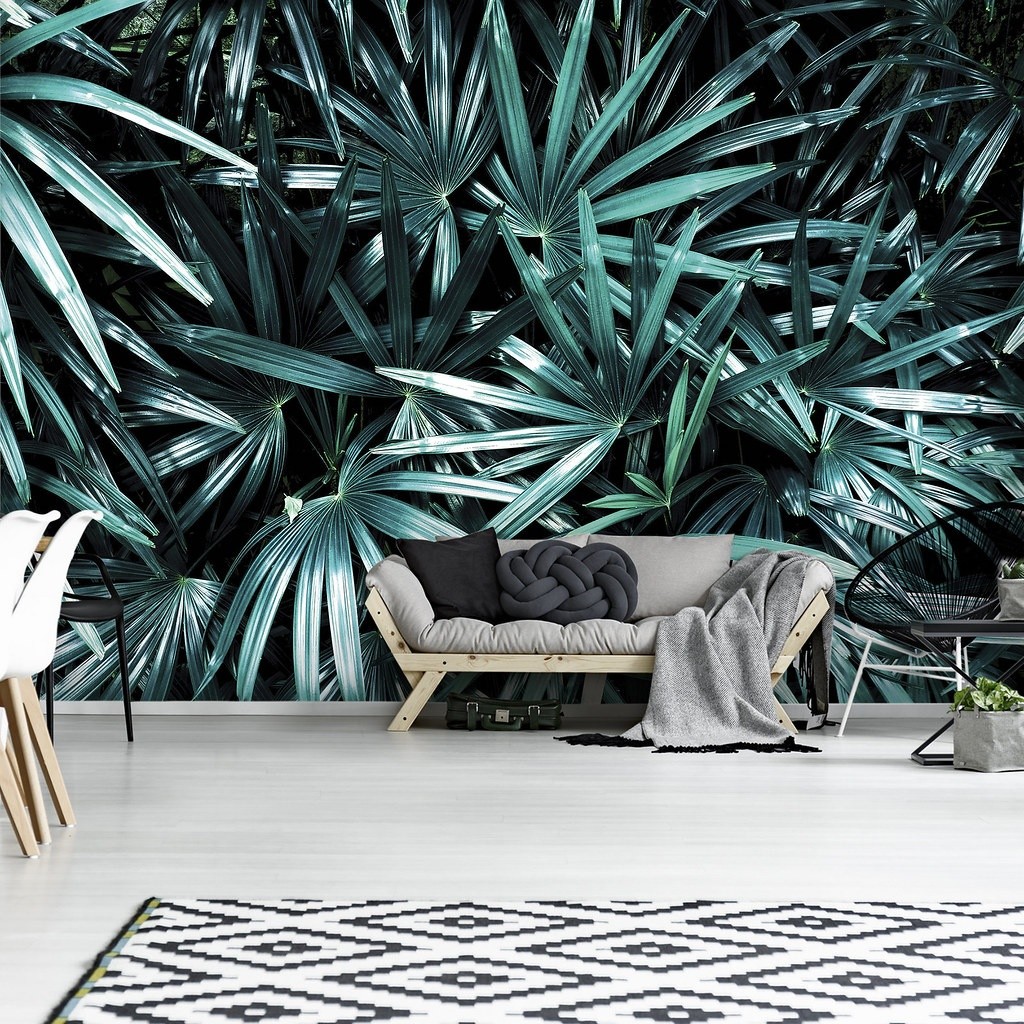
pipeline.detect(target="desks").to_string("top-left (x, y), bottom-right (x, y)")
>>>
top-left (909, 618), bottom-right (1024, 766)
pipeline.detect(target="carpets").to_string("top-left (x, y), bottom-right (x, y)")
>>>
top-left (42, 897), bottom-right (1024, 1024)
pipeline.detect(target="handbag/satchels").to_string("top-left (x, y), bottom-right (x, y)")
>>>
top-left (993, 561), bottom-right (1024, 621)
top-left (953, 687), bottom-right (1024, 772)
top-left (445, 692), bottom-right (564, 731)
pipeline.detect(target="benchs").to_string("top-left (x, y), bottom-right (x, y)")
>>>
top-left (362, 552), bottom-right (834, 736)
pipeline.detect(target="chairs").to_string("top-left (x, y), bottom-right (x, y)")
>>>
top-left (837, 500), bottom-right (1024, 737)
top-left (29, 551), bottom-right (136, 741)
top-left (1, 506), bottom-right (104, 859)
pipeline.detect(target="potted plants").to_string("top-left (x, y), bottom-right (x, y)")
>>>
top-left (996, 559), bottom-right (1024, 622)
top-left (946, 676), bottom-right (1024, 773)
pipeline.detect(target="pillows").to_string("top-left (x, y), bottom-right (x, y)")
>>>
top-left (395, 525), bottom-right (514, 625)
top-left (584, 531), bottom-right (736, 619)
top-left (433, 531), bottom-right (589, 559)
